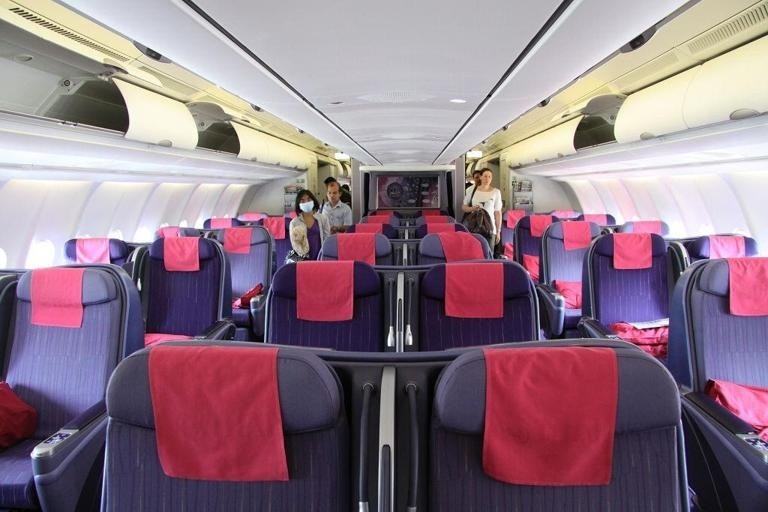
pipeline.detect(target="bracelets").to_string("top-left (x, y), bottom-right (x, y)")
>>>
top-left (496, 228), bottom-right (501, 232)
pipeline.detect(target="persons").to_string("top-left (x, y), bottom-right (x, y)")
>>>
top-left (289, 189), bottom-right (331, 262)
top-left (473, 170), bottom-right (483, 185)
top-left (319, 177), bottom-right (352, 211)
top-left (342, 184), bottom-right (352, 196)
top-left (461, 168), bottom-right (502, 261)
top-left (320, 183), bottom-right (354, 235)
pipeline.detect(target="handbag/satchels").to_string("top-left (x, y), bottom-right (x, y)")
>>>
top-left (462, 212), bottom-right (471, 224)
top-left (464, 208), bottom-right (493, 239)
top-left (284, 250), bottom-right (304, 264)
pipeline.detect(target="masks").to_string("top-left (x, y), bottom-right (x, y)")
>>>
top-left (299, 201), bottom-right (314, 212)
